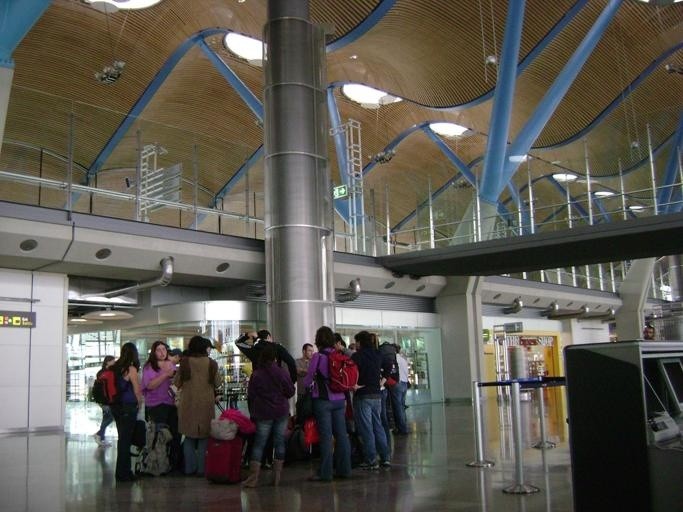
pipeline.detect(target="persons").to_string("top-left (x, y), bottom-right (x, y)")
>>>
top-left (139, 323), bottom-right (412, 490)
top-left (91, 353), bottom-right (117, 448)
top-left (107, 341), bottom-right (143, 484)
top-left (641, 325), bottom-right (656, 340)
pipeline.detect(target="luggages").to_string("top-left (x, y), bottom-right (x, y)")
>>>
top-left (204, 435), bottom-right (243, 483)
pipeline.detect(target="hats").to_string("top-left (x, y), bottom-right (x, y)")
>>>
top-left (204, 338), bottom-right (216, 348)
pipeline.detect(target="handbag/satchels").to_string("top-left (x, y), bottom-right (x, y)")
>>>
top-left (210, 408), bottom-right (256, 440)
top-left (284, 392), bottom-right (320, 467)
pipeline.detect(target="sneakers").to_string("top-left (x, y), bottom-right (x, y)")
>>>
top-left (116, 468), bottom-right (137, 481)
top-left (93, 434), bottom-right (112, 446)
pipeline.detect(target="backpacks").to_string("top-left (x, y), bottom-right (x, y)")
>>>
top-left (92, 370), bottom-right (117, 404)
top-left (332, 431), bottom-right (361, 470)
top-left (316, 350), bottom-right (359, 400)
top-left (128, 420), bottom-right (173, 476)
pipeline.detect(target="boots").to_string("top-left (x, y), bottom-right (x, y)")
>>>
top-left (240, 460), bottom-right (261, 488)
top-left (272, 459), bottom-right (284, 487)
